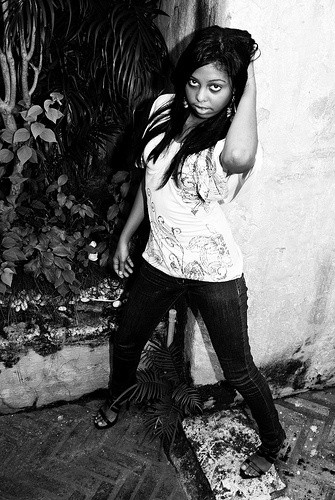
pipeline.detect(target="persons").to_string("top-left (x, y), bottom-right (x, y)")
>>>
top-left (92, 25), bottom-right (289, 478)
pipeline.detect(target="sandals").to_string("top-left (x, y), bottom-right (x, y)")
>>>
top-left (239, 437), bottom-right (284, 479)
top-left (94, 394), bottom-right (135, 431)
